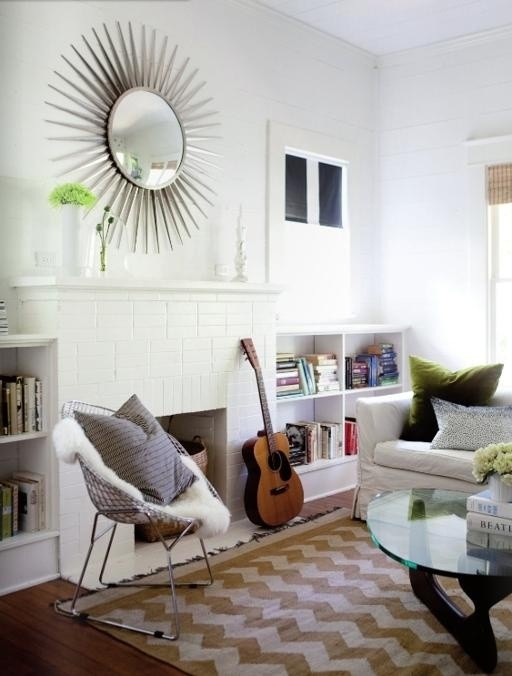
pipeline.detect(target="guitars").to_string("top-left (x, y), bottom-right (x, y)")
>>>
top-left (240, 338), bottom-right (304, 526)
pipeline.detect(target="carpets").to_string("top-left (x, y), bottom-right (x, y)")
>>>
top-left (55, 502), bottom-right (512, 676)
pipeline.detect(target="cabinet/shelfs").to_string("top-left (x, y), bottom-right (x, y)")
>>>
top-left (275, 327), bottom-right (404, 501)
top-left (1, 337), bottom-right (63, 596)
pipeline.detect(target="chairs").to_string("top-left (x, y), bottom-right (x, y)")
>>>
top-left (58, 400), bottom-right (228, 640)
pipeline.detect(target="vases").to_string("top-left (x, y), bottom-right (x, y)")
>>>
top-left (487, 476), bottom-right (511, 502)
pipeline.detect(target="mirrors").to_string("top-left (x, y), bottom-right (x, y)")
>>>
top-left (43, 21), bottom-right (225, 256)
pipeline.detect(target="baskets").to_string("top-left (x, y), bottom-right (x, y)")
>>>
top-left (138, 435), bottom-right (208, 543)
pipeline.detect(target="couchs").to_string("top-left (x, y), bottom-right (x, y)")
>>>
top-left (351, 394), bottom-right (512, 524)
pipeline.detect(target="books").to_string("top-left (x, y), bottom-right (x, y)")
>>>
top-left (466, 511), bottom-right (511, 539)
top-left (466, 542), bottom-right (511, 569)
top-left (466, 530), bottom-right (512, 555)
top-left (275, 343), bottom-right (401, 466)
top-left (466, 486), bottom-right (512, 520)
top-left (0, 300), bottom-right (45, 543)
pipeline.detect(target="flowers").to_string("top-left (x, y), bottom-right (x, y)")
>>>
top-left (472, 442), bottom-right (512, 484)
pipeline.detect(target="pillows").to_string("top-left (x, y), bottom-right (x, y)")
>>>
top-left (428, 397), bottom-right (512, 450)
top-left (71, 394), bottom-right (198, 504)
top-left (400, 354), bottom-right (504, 442)
